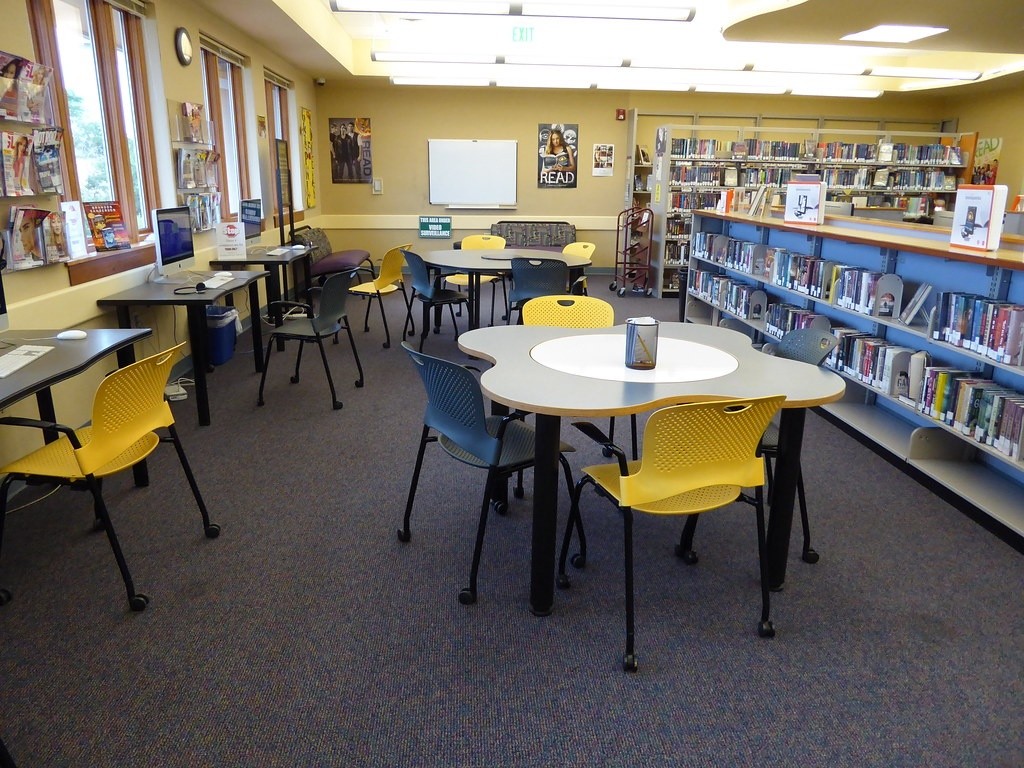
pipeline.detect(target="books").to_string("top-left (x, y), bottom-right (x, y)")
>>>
top-left (0, 49), bottom-right (68, 269)
top-left (543, 152), bottom-right (568, 169)
top-left (633, 144), bottom-right (653, 216)
top-left (83, 201), bottom-right (132, 252)
top-left (178, 102), bottom-right (222, 231)
top-left (949, 184), bottom-right (1008, 252)
top-left (784, 180), bottom-right (827, 226)
top-left (664, 136), bottom-right (962, 290)
top-left (688, 231), bottom-right (1024, 462)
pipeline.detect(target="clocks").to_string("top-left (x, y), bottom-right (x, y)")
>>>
top-left (176, 27), bottom-right (193, 66)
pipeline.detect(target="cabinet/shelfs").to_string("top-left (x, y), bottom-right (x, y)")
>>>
top-left (609, 108), bottom-right (1024, 554)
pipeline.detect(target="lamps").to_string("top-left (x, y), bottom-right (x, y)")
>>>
top-left (330, 0), bottom-right (987, 99)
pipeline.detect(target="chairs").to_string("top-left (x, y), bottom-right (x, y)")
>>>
top-left (333, 234), bottom-right (842, 673)
top-left (256, 267), bottom-right (364, 410)
top-left (0, 340), bottom-right (221, 612)
top-left (291, 226), bottom-right (376, 301)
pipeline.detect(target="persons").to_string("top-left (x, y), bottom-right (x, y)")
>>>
top-left (329, 122), bottom-right (363, 179)
top-left (1, 59), bottom-right (22, 98)
top-left (542, 130), bottom-right (575, 180)
top-left (27, 65), bottom-right (46, 112)
top-left (18, 210), bottom-right (41, 257)
top-left (12, 135), bottom-right (29, 191)
top-left (50, 212), bottom-right (68, 257)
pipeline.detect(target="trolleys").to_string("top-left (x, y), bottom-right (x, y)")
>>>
top-left (609, 208), bottom-right (654, 298)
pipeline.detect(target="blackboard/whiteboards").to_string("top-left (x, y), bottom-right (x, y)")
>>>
top-left (428, 138), bottom-right (518, 209)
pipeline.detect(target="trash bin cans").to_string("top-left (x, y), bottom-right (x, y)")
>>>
top-left (206, 305), bottom-right (235, 365)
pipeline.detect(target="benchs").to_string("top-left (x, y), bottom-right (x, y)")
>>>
top-left (489, 221), bottom-right (575, 278)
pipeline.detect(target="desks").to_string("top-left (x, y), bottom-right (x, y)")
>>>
top-left (458, 322), bottom-right (847, 617)
top-left (97, 270), bottom-right (270, 426)
top-left (209, 246), bottom-right (319, 351)
top-left (0, 328), bottom-right (151, 488)
top-left (420, 248), bottom-right (591, 360)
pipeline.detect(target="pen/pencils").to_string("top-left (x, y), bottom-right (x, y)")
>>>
top-left (637, 333), bottom-right (654, 366)
